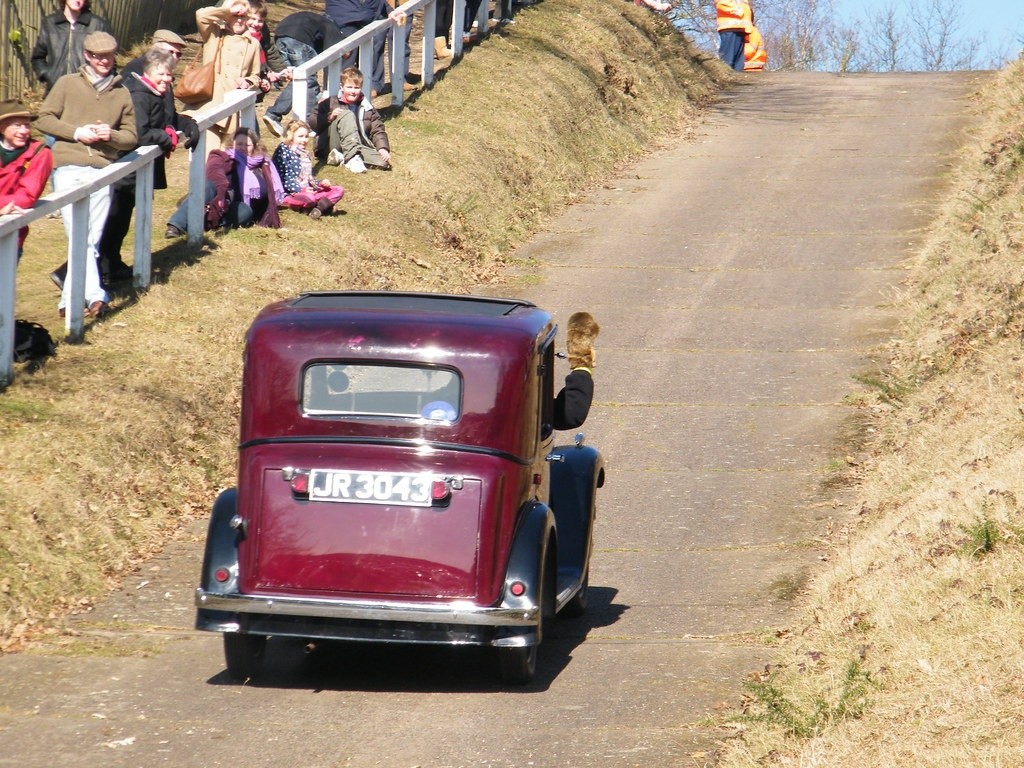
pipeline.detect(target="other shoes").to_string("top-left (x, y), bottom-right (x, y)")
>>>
top-left (263, 116), bottom-right (284, 136)
top-left (102, 267), bottom-right (133, 285)
top-left (51, 261), bottom-right (68, 289)
top-left (403, 80), bottom-right (418, 90)
top-left (492, 18), bottom-right (515, 24)
top-left (89, 302), bottom-right (110, 317)
top-left (165, 224), bottom-right (180, 239)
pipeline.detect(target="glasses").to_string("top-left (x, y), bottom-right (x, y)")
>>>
top-left (171, 51), bottom-right (182, 58)
top-left (93, 54), bottom-right (117, 59)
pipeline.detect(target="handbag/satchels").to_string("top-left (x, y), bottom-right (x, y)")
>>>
top-left (174, 29), bottom-right (225, 104)
top-left (14, 319), bottom-right (54, 362)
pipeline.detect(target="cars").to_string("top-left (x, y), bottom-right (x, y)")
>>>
top-left (194, 289), bottom-right (606, 680)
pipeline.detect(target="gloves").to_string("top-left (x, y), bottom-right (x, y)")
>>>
top-left (184, 124), bottom-right (200, 152)
top-left (160, 135), bottom-right (172, 151)
top-left (165, 127), bottom-right (178, 152)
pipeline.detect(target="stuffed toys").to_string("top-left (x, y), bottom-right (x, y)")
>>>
top-left (566, 312), bottom-right (600, 369)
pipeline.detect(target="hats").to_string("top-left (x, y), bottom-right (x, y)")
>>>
top-left (0, 100), bottom-right (39, 121)
top-left (84, 31), bottom-right (117, 53)
top-left (153, 29), bottom-right (188, 48)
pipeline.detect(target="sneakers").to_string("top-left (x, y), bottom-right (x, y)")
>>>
top-left (345, 154), bottom-right (368, 174)
top-left (327, 149), bottom-right (343, 165)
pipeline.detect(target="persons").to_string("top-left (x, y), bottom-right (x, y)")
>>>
top-left (43, 29), bottom-right (201, 294)
top-left (714, 0), bottom-right (769, 73)
top-left (34, 30), bottom-right (138, 320)
top-left (318, 66), bottom-right (391, 174)
top-left (321, 0), bottom-right (408, 89)
top-left (371, 0), bottom-right (425, 98)
top-left (162, 126), bottom-right (285, 237)
top-left (30, 0), bottom-right (114, 219)
top-left (430, 0), bottom-right (482, 60)
top-left (432, 336), bottom-right (596, 443)
top-left (272, 119), bottom-right (345, 220)
top-left (0, 98), bottom-right (53, 270)
top-left (263, 12), bottom-right (353, 139)
top-left (182, 0), bottom-right (295, 152)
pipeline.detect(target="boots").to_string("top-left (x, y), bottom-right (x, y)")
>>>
top-left (435, 36), bottom-right (452, 59)
top-left (301, 196), bottom-right (333, 218)
